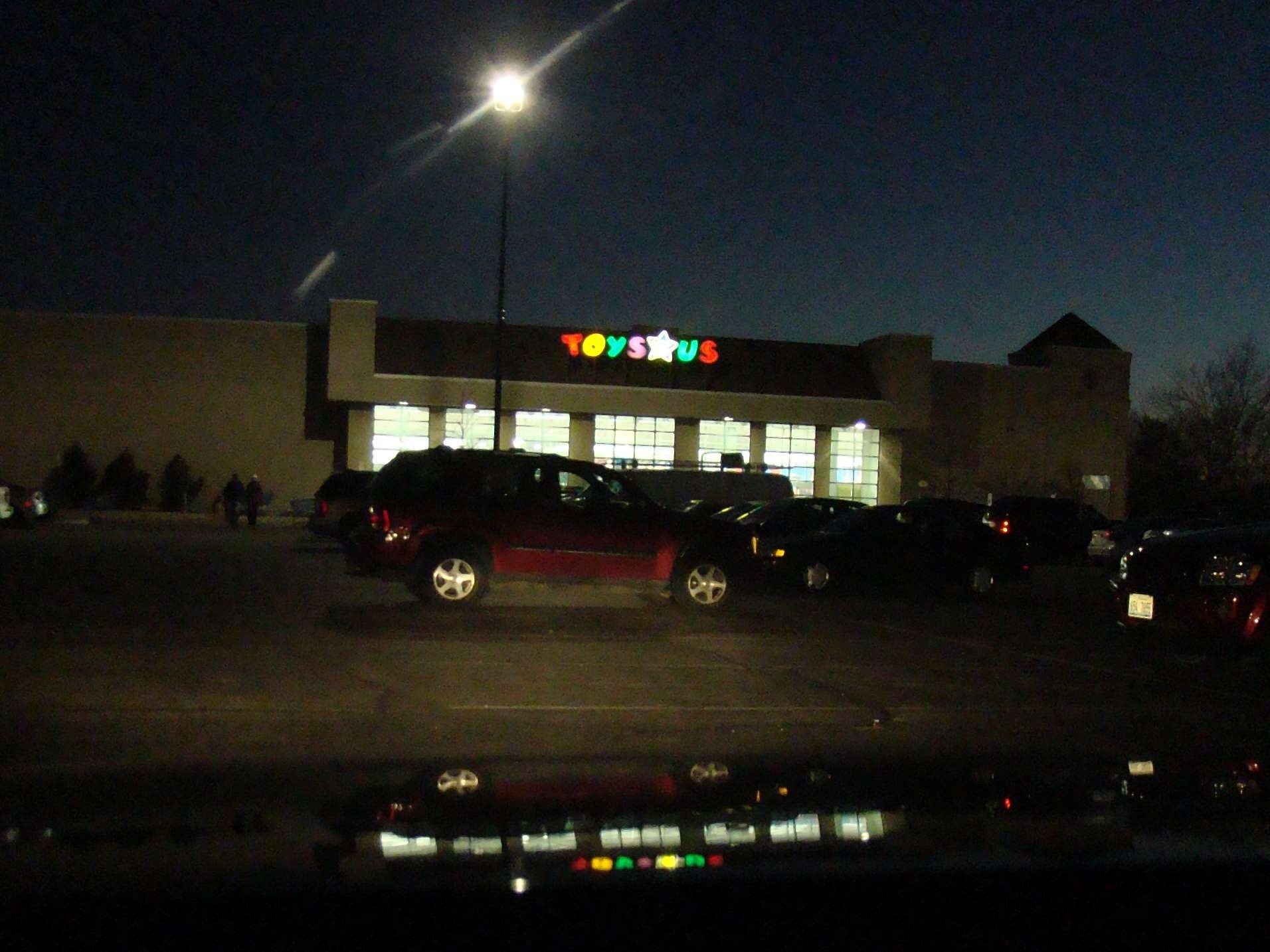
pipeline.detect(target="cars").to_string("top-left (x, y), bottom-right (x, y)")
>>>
top-left (678, 493), bottom-right (1270, 670)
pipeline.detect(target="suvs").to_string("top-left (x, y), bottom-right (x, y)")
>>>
top-left (358, 447), bottom-right (753, 614)
top-left (308, 468), bottom-right (380, 546)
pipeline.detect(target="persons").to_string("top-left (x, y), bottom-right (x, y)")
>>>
top-left (223, 473), bottom-right (247, 529)
top-left (243, 473), bottom-right (265, 526)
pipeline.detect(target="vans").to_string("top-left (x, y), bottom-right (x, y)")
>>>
top-left (577, 467), bottom-right (794, 516)
